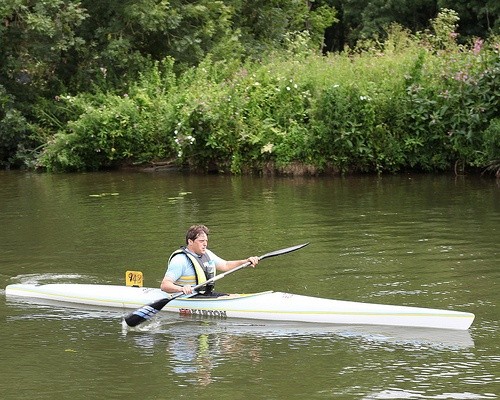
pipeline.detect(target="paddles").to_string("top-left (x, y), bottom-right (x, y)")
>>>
top-left (126, 241), bottom-right (309, 324)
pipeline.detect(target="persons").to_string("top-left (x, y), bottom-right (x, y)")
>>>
top-left (160, 224), bottom-right (260, 299)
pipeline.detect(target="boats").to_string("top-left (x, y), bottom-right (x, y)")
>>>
top-left (6, 282), bottom-right (475, 332)
